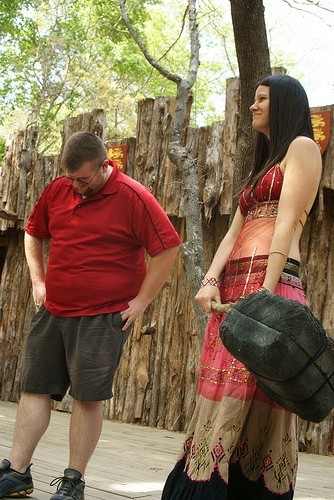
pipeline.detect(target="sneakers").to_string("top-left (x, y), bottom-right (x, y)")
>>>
top-left (0, 459), bottom-right (34, 500)
top-left (50, 468), bottom-right (86, 500)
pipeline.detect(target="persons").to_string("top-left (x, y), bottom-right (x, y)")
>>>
top-left (0, 129), bottom-right (183, 500)
top-left (160, 75), bottom-right (323, 500)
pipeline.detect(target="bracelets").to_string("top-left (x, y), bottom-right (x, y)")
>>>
top-left (267, 250), bottom-right (287, 257)
top-left (200, 277), bottom-right (222, 288)
top-left (261, 285), bottom-right (275, 296)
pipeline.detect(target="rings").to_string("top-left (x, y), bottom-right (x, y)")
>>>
top-left (36, 304), bottom-right (39, 307)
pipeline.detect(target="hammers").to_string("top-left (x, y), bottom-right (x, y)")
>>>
top-left (211, 291), bottom-right (334, 423)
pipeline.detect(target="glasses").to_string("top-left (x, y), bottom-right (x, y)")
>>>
top-left (63, 158), bottom-right (106, 186)
